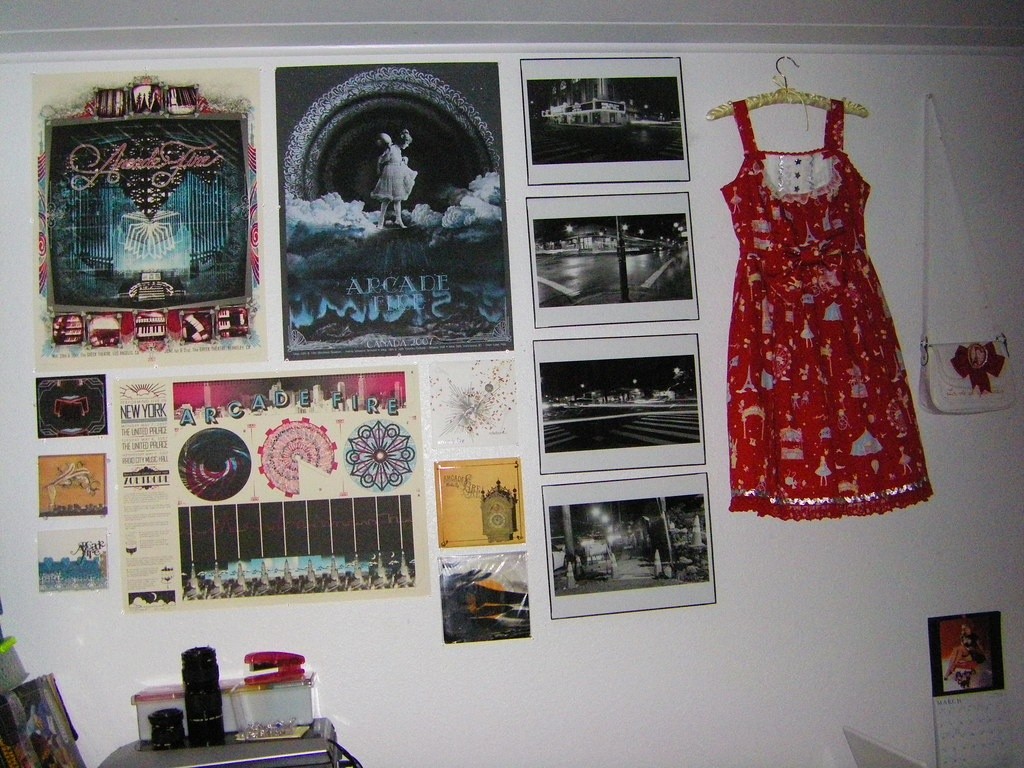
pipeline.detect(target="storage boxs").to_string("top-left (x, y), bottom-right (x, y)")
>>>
top-left (132, 673), bottom-right (316, 744)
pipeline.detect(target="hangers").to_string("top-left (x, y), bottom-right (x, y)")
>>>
top-left (706, 57), bottom-right (868, 121)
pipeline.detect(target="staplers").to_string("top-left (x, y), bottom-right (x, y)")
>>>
top-left (244, 652), bottom-right (306, 685)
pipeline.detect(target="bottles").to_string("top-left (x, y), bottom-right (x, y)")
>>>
top-left (181, 646), bottom-right (226, 748)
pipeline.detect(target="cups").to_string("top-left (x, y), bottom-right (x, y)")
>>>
top-left (147, 707), bottom-right (186, 751)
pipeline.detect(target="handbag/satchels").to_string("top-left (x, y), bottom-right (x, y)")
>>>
top-left (917, 337), bottom-right (1016, 415)
